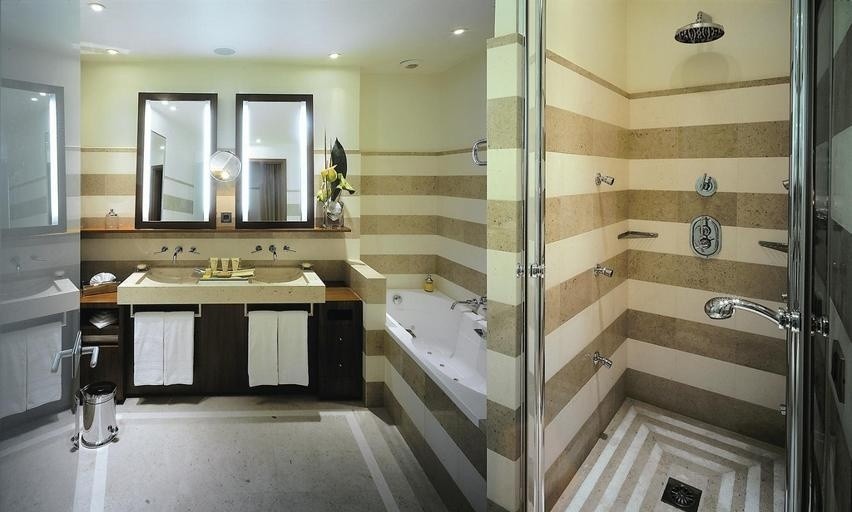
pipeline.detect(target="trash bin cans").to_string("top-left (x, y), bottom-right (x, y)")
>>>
top-left (80, 381), bottom-right (119, 447)
top-left (70, 391), bottom-right (81, 452)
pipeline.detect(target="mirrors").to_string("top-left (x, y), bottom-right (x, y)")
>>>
top-left (208, 150), bottom-right (242, 184)
top-left (0, 77), bottom-right (67, 241)
top-left (235, 92), bottom-right (316, 229)
top-left (134, 90), bottom-right (219, 229)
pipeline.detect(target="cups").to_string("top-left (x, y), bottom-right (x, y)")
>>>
top-left (210, 257), bottom-right (218, 271)
top-left (221, 258), bottom-right (229, 272)
top-left (232, 258), bottom-right (239, 271)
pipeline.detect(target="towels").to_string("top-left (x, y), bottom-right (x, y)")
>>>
top-left (26, 322), bottom-right (62, 414)
top-left (248, 310), bottom-right (278, 389)
top-left (278, 310), bottom-right (310, 388)
top-left (0, 328), bottom-right (27, 423)
top-left (164, 311), bottom-right (195, 388)
top-left (133, 311), bottom-right (164, 387)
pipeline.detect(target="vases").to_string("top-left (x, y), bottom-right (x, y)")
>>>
top-left (322, 203), bottom-right (345, 230)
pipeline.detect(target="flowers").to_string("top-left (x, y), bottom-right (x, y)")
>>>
top-left (316, 127), bottom-right (356, 221)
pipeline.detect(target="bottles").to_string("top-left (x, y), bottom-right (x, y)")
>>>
top-left (425, 274), bottom-right (434, 292)
top-left (105, 209), bottom-right (121, 229)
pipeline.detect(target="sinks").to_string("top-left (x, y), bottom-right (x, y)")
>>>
top-left (249, 266), bottom-right (308, 286)
top-left (135, 266), bottom-right (202, 285)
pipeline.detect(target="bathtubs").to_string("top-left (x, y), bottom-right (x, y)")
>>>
top-left (385, 287), bottom-right (487, 431)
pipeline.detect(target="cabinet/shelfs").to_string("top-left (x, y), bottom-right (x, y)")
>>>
top-left (80, 292), bottom-right (126, 406)
top-left (312, 286), bottom-right (363, 403)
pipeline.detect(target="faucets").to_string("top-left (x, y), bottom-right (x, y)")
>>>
top-left (170, 245), bottom-right (183, 265)
top-left (269, 245), bottom-right (280, 263)
top-left (449, 296), bottom-right (487, 315)
